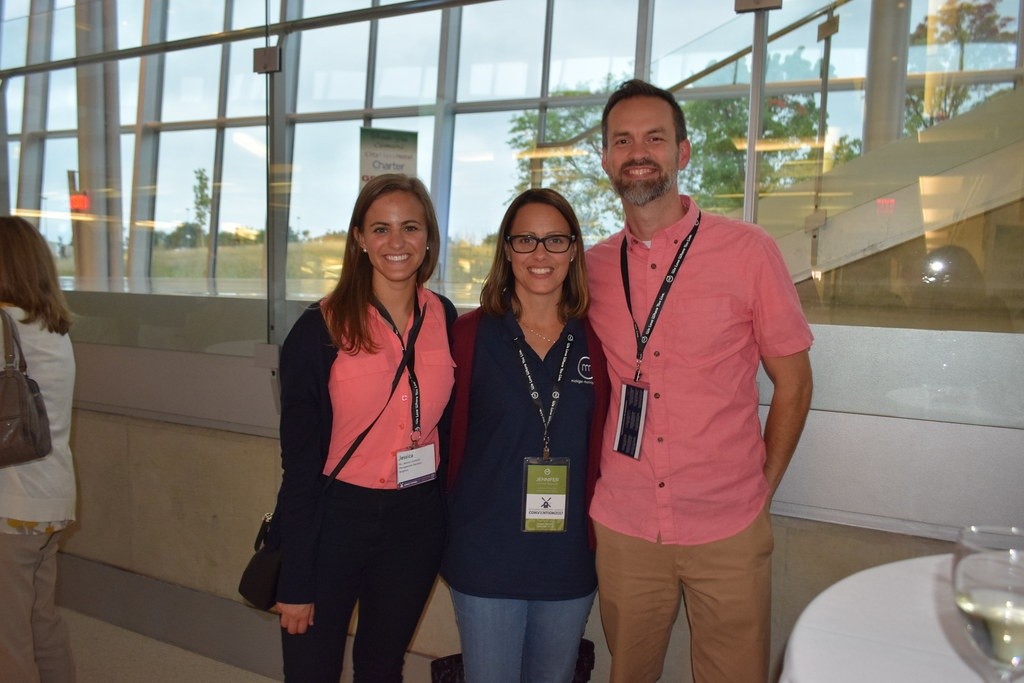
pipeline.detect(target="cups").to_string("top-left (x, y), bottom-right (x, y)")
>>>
top-left (951, 525), bottom-right (1024, 683)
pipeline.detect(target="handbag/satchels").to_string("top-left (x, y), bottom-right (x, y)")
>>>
top-left (237, 512), bottom-right (288, 611)
top-left (0, 308), bottom-right (52, 471)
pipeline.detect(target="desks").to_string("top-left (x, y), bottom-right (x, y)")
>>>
top-left (777, 550), bottom-right (1024, 683)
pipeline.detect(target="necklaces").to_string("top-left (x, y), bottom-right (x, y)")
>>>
top-left (515, 316), bottom-right (556, 344)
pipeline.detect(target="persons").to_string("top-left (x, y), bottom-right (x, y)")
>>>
top-left (450, 187), bottom-right (611, 683)
top-left (578, 79), bottom-right (813, 682)
top-left (0, 215), bottom-right (81, 683)
top-left (278, 172), bottom-right (460, 683)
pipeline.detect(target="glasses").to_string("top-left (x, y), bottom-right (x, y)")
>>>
top-left (505, 234), bottom-right (576, 254)
